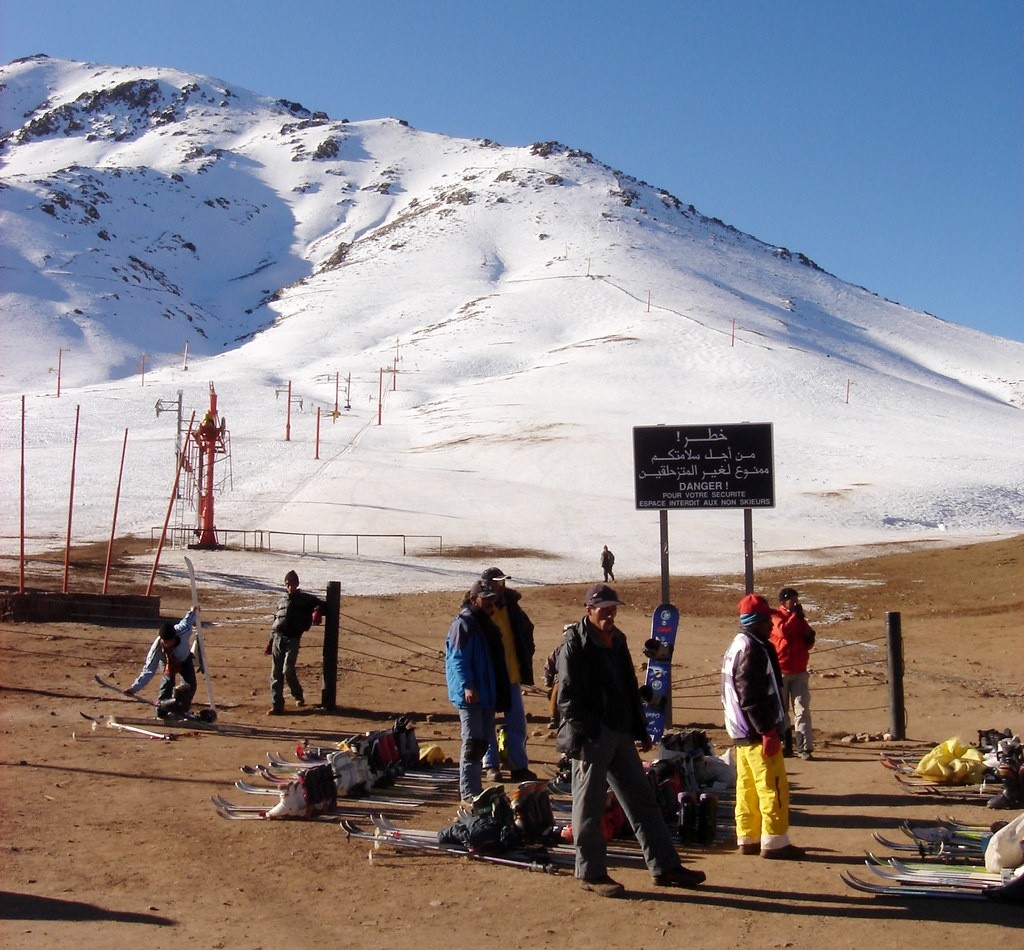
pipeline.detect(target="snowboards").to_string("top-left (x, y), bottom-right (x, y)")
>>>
top-left (640, 603), bottom-right (685, 742)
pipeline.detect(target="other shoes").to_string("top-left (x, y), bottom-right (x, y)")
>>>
top-left (580, 872), bottom-right (628, 897)
top-left (781, 748), bottom-right (796, 757)
top-left (759, 842), bottom-right (807, 860)
top-left (266, 707), bottom-right (283, 716)
top-left (511, 769), bottom-right (538, 782)
top-left (739, 840), bottom-right (766, 855)
top-left (796, 751), bottom-right (813, 761)
top-left (295, 699), bottom-right (304, 707)
top-left (547, 718), bottom-right (560, 729)
top-left (650, 863), bottom-right (708, 887)
top-left (460, 795), bottom-right (476, 808)
top-left (487, 767), bottom-right (507, 782)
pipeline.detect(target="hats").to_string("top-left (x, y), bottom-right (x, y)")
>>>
top-left (482, 566), bottom-right (513, 583)
top-left (158, 620), bottom-right (176, 640)
top-left (283, 569), bottom-right (301, 585)
top-left (583, 583), bottom-right (627, 609)
top-left (469, 580), bottom-right (498, 597)
top-left (738, 595), bottom-right (780, 617)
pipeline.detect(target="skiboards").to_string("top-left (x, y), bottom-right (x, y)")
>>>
top-left (79, 557), bottom-right (217, 740)
top-left (837, 743), bottom-right (1024, 902)
top-left (504, 747), bottom-right (737, 825)
top-left (209, 739), bottom-right (460, 825)
top-left (335, 814), bottom-right (680, 872)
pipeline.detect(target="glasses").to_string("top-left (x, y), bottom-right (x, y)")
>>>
top-left (783, 590), bottom-right (797, 599)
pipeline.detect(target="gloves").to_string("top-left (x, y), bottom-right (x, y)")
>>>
top-left (263, 641), bottom-right (277, 656)
top-left (763, 727), bottom-right (785, 759)
top-left (805, 631), bottom-right (816, 644)
top-left (793, 603), bottom-right (804, 616)
top-left (312, 609), bottom-right (323, 628)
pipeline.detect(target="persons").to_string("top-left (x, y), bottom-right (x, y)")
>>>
top-left (768, 588), bottom-right (817, 761)
top-left (600, 545), bottom-right (615, 582)
top-left (722, 594), bottom-right (805, 858)
top-left (125, 605), bottom-right (201, 720)
top-left (264, 570), bottom-right (323, 716)
top-left (544, 623), bottom-right (577, 729)
top-left (481, 567), bottom-right (538, 781)
top-left (445, 584), bottom-right (497, 807)
top-left (556, 584), bottom-right (708, 897)
